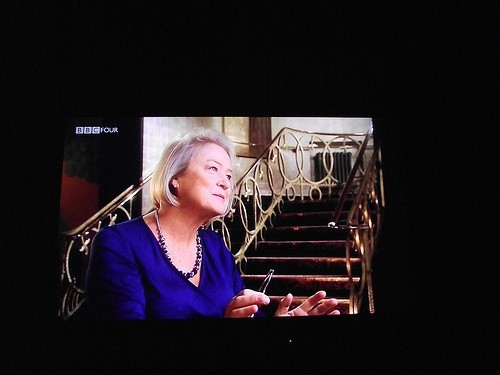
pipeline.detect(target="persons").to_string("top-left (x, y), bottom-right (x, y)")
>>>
top-left (81, 131), bottom-right (341, 317)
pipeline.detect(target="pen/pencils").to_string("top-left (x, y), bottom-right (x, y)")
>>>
top-left (249, 269), bottom-right (275, 316)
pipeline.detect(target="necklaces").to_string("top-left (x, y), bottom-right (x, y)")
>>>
top-left (155, 208), bottom-right (203, 279)
